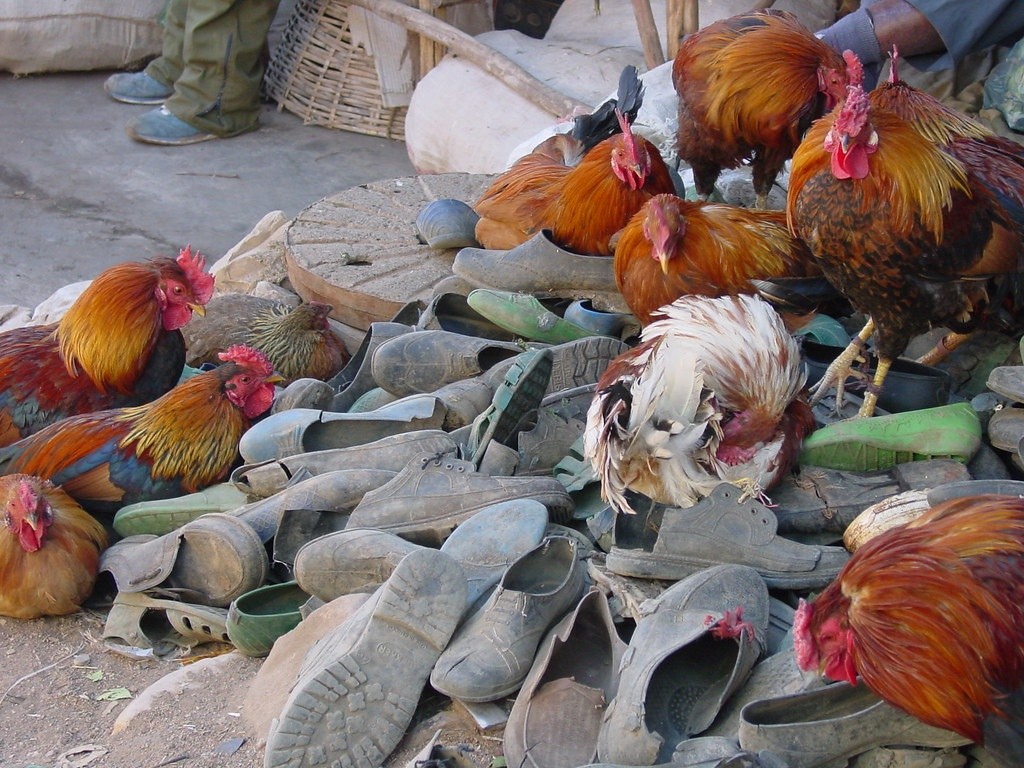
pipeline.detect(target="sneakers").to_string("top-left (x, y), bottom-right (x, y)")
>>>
top-left (126, 104), bottom-right (218, 146)
top-left (104, 69), bottom-right (176, 105)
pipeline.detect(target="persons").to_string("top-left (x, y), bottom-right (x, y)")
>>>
top-left (103, 0), bottom-right (281, 145)
top-left (810, 0), bottom-right (1024, 92)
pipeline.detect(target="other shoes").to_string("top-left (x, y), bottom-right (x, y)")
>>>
top-left (95, 197), bottom-right (1024, 768)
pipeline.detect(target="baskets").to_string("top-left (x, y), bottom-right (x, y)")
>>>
top-left (259, 0), bottom-right (409, 142)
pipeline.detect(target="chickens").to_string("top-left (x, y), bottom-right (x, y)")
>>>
top-left (791, 492), bottom-right (1024, 768)
top-left (475, 7), bottom-right (1024, 517)
top-left (0, 243), bottom-right (353, 619)
